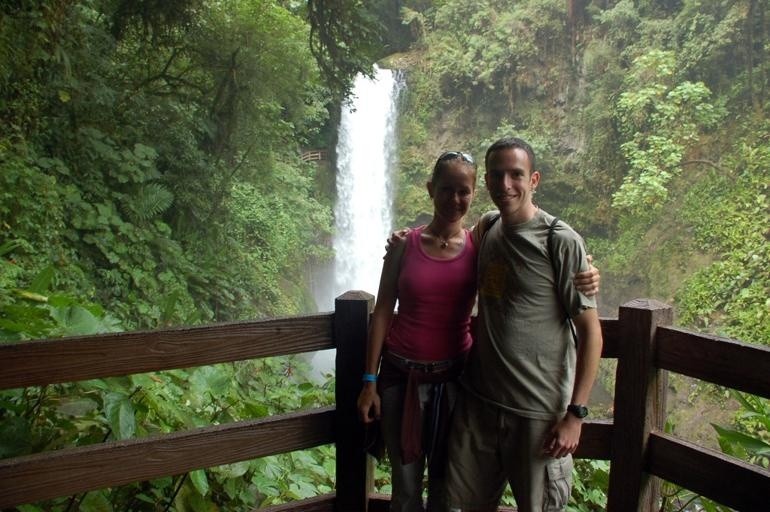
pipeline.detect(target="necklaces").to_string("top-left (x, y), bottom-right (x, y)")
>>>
top-left (432, 230), bottom-right (459, 250)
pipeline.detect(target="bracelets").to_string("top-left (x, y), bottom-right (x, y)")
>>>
top-left (362, 374), bottom-right (377, 381)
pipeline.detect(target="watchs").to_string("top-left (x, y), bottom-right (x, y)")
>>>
top-left (566, 404), bottom-right (589, 420)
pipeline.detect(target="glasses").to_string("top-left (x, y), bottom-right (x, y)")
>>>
top-left (434, 152), bottom-right (473, 164)
